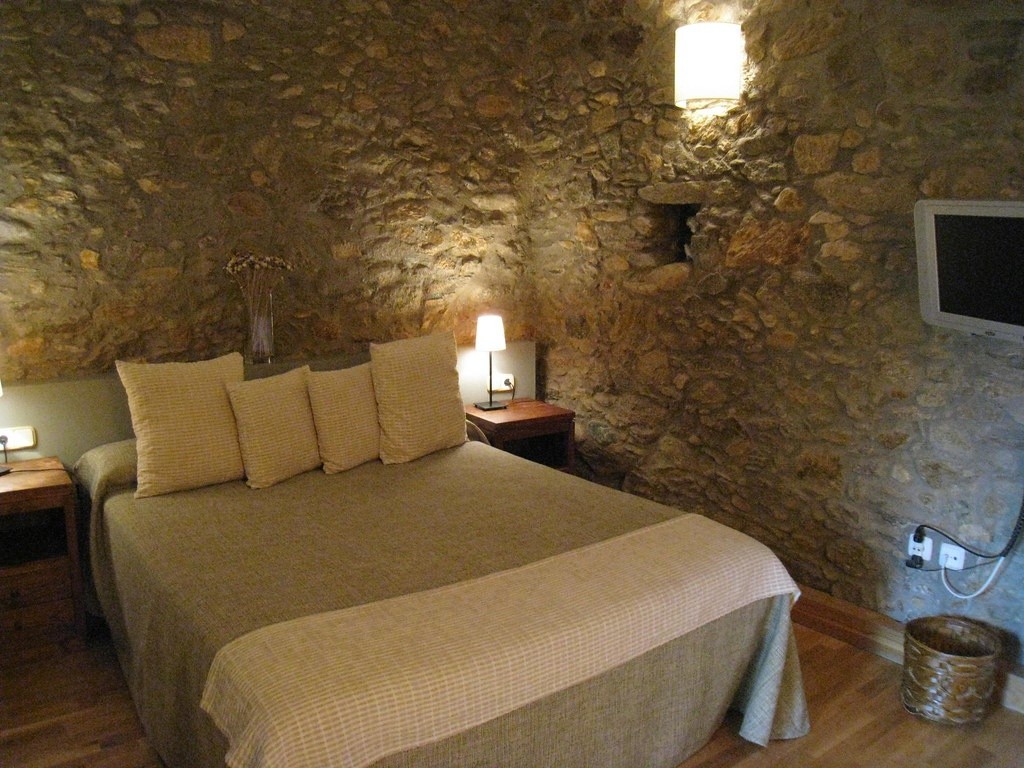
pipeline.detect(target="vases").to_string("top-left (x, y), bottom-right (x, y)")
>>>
top-left (900, 619), bottom-right (1002, 727)
top-left (242, 294), bottom-right (276, 363)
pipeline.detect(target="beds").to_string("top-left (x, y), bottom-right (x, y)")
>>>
top-left (68, 333), bottom-right (810, 768)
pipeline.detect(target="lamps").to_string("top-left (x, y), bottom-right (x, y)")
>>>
top-left (673, 22), bottom-right (745, 111)
top-left (474, 315), bottom-right (508, 411)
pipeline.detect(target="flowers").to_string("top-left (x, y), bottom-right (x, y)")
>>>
top-left (222, 248), bottom-right (293, 357)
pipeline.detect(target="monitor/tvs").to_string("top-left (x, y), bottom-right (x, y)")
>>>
top-left (912, 200), bottom-right (1024, 343)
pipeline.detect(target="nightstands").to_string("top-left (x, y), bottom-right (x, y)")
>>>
top-left (0, 459), bottom-right (86, 650)
top-left (465, 398), bottom-right (575, 477)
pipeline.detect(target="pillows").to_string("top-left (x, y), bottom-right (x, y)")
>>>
top-left (370, 330), bottom-right (470, 466)
top-left (304, 361), bottom-right (381, 475)
top-left (226, 365), bottom-right (322, 489)
top-left (115, 351), bottom-right (246, 499)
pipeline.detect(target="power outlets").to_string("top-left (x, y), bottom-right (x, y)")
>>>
top-left (908, 534), bottom-right (932, 562)
top-left (0, 426), bottom-right (37, 451)
top-left (937, 544), bottom-right (965, 572)
top-left (487, 373), bottom-right (515, 391)
